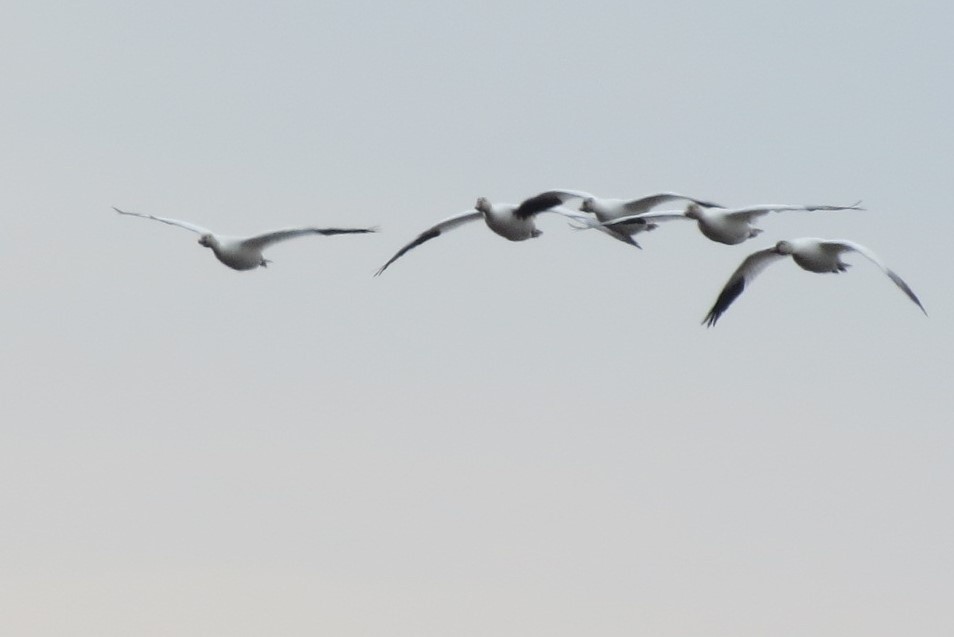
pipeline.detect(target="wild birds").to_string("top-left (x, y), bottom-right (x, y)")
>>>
top-left (699, 238), bottom-right (930, 328)
top-left (599, 199), bottom-right (866, 246)
top-left (112, 207), bottom-right (378, 272)
top-left (373, 189), bottom-right (643, 277)
top-left (510, 189), bottom-right (727, 234)
